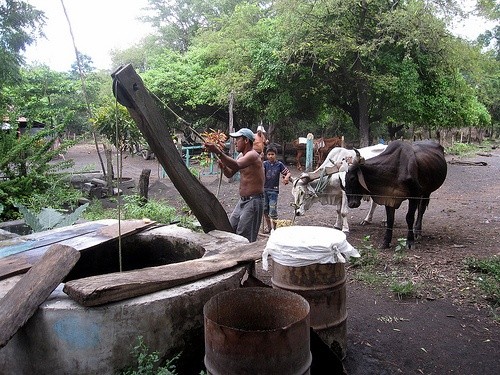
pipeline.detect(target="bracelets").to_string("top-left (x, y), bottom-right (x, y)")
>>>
top-left (218, 150), bottom-right (223, 156)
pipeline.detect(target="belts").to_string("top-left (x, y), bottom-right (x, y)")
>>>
top-left (241, 195), bottom-right (264, 201)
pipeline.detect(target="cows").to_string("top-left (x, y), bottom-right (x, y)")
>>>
top-left (283, 139), bottom-right (447, 250)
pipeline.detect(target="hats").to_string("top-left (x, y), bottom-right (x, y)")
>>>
top-left (257, 126), bottom-right (266, 133)
top-left (229, 129), bottom-right (255, 142)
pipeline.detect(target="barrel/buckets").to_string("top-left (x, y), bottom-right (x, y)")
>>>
top-left (204, 286), bottom-right (312, 375)
top-left (271, 226), bottom-right (348, 360)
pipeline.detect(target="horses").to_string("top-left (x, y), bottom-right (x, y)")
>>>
top-left (292, 136), bottom-right (349, 171)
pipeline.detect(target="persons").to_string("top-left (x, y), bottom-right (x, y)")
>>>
top-left (204, 128), bottom-right (265, 242)
top-left (376, 134), bottom-right (386, 145)
top-left (254, 126), bottom-right (291, 233)
